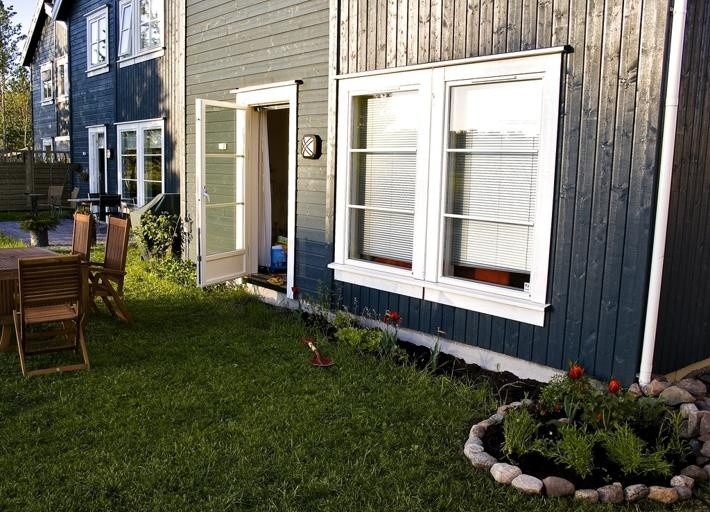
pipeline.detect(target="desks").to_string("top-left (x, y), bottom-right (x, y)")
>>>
top-left (0, 244), bottom-right (64, 353)
top-left (24, 193), bottom-right (48, 214)
top-left (67, 197), bottom-right (100, 213)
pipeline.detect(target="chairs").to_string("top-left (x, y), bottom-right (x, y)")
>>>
top-left (37, 185), bottom-right (80, 216)
top-left (88, 192), bottom-right (124, 233)
top-left (9, 210), bottom-right (133, 378)
